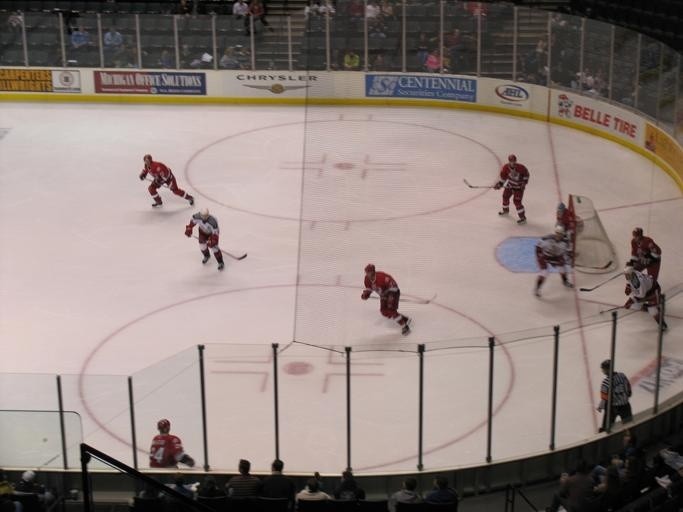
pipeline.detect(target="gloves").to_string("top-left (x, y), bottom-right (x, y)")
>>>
top-left (139, 172), bottom-right (146, 180)
top-left (493, 183), bottom-right (501, 190)
top-left (566, 229), bottom-right (574, 240)
top-left (387, 295), bottom-right (395, 309)
top-left (520, 182), bottom-right (526, 190)
top-left (624, 297), bottom-right (634, 310)
top-left (644, 253), bottom-right (653, 259)
top-left (595, 406), bottom-right (604, 412)
top-left (541, 263), bottom-right (555, 274)
top-left (562, 263), bottom-right (573, 274)
top-left (210, 234), bottom-right (218, 248)
top-left (626, 259), bottom-right (637, 266)
top-left (624, 284), bottom-right (632, 296)
top-left (185, 225), bottom-right (192, 237)
top-left (361, 290), bottom-right (371, 300)
top-left (151, 180), bottom-right (161, 188)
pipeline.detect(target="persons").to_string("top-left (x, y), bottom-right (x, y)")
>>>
top-left (70, 24), bottom-right (93, 47)
top-left (520, 14), bottom-right (645, 109)
top-left (389, 477), bottom-right (423, 511)
top-left (139, 478), bottom-right (166, 500)
top-left (334, 471), bottom-right (366, 501)
top-left (233, 0), bottom-right (250, 37)
top-left (534, 226), bottom-right (575, 298)
top-left (259, 460), bottom-right (294, 498)
top-left (103, 24), bottom-right (123, 47)
top-left (14, 470), bottom-right (47, 494)
top-left (198, 476), bottom-right (224, 496)
top-left (172, 478), bottom-right (194, 500)
top-left (225, 460), bottom-right (262, 497)
top-left (494, 154), bottom-right (530, 223)
top-left (186, 207), bottom-right (223, 270)
top-left (413, 1), bottom-right (489, 74)
top-left (249, 1), bottom-right (275, 33)
top-left (424, 476), bottom-right (463, 502)
top-left (619, 459), bottom-right (639, 483)
top-left (343, 50), bottom-right (361, 69)
top-left (556, 202), bottom-right (582, 231)
top-left (140, 154), bottom-right (194, 208)
top-left (374, 51), bottom-right (397, 71)
top-left (593, 463), bottom-right (622, 493)
top-left (629, 228), bottom-right (662, 281)
top-left (220, 44), bottom-right (249, 69)
top-left (595, 360), bottom-right (633, 432)
top-left (148, 419), bottom-right (194, 470)
top-left (360, 264), bottom-right (412, 336)
top-left (303, 0), bottom-right (403, 42)
top-left (544, 471), bottom-right (593, 512)
top-left (622, 435), bottom-right (635, 459)
top-left (0, 468), bottom-right (14, 495)
top-left (296, 478), bottom-right (331, 501)
top-left (624, 266), bottom-right (668, 332)
top-left (159, 38), bottom-right (250, 71)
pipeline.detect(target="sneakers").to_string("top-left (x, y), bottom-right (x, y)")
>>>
top-left (563, 282), bottom-right (574, 288)
top-left (401, 325), bottom-right (409, 334)
top-left (202, 255), bottom-right (210, 264)
top-left (661, 323), bottom-right (667, 332)
top-left (533, 291), bottom-right (541, 297)
top-left (517, 217), bottom-right (526, 223)
top-left (218, 262), bottom-right (224, 270)
top-left (152, 202), bottom-right (162, 206)
top-left (498, 210), bottom-right (509, 215)
top-left (190, 198), bottom-right (193, 205)
top-left (403, 316), bottom-right (408, 323)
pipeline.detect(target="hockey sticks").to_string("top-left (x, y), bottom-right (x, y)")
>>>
top-left (579, 271), bottom-right (626, 294)
top-left (190, 233), bottom-right (248, 261)
top-left (368, 292), bottom-right (437, 305)
top-left (599, 306), bottom-right (626, 316)
top-left (544, 257), bottom-right (614, 271)
top-left (463, 178), bottom-right (496, 191)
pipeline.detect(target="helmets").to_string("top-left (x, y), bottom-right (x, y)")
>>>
top-left (600, 359), bottom-right (611, 369)
top-left (143, 155), bottom-right (152, 162)
top-left (508, 155), bottom-right (517, 163)
top-left (200, 208), bottom-right (209, 216)
top-left (364, 264), bottom-right (375, 272)
top-left (554, 225), bottom-right (566, 235)
top-left (157, 419), bottom-right (170, 430)
top-left (632, 227), bottom-right (643, 237)
top-left (557, 202), bottom-right (565, 210)
top-left (624, 266), bottom-right (634, 274)
